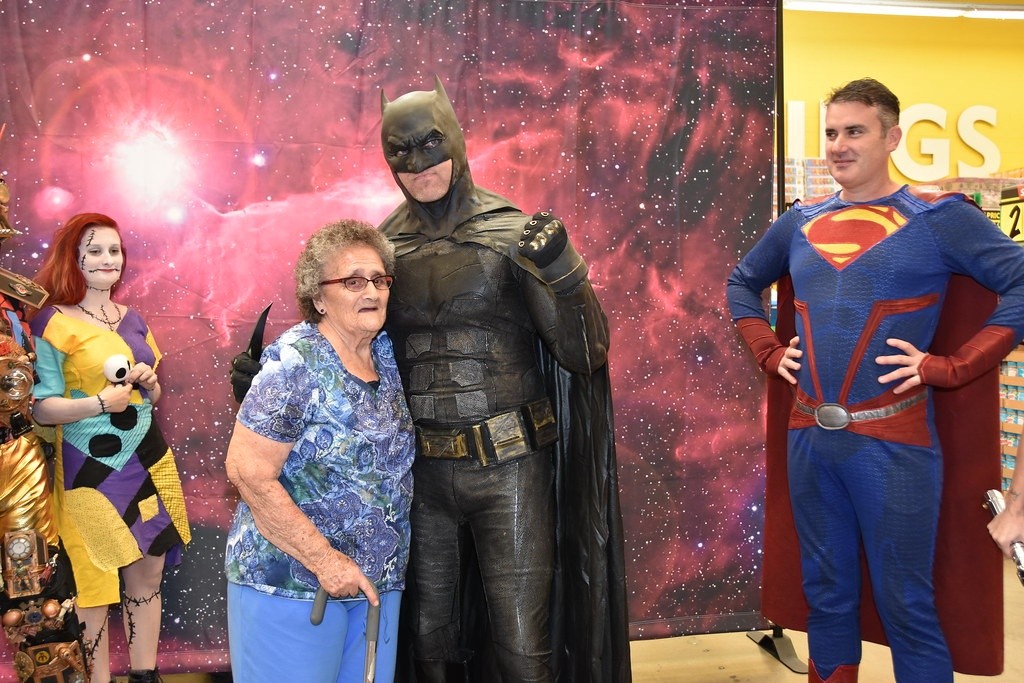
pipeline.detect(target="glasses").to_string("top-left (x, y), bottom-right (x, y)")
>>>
top-left (319, 275), bottom-right (395, 293)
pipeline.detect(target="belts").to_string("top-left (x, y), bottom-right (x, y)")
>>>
top-left (415, 396), bottom-right (562, 467)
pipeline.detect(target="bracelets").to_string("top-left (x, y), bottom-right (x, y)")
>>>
top-left (146, 386), bottom-right (156, 392)
top-left (96, 394), bottom-right (111, 414)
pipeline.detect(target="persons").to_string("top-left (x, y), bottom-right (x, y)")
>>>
top-left (31, 213), bottom-right (192, 683)
top-left (725, 78), bottom-right (1024, 683)
top-left (225, 72), bottom-right (633, 683)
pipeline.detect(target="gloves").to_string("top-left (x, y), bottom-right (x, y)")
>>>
top-left (228, 353), bottom-right (262, 403)
top-left (518, 212), bottom-right (589, 293)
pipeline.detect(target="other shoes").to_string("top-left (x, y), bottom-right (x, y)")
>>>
top-left (128, 666), bottom-right (163, 683)
